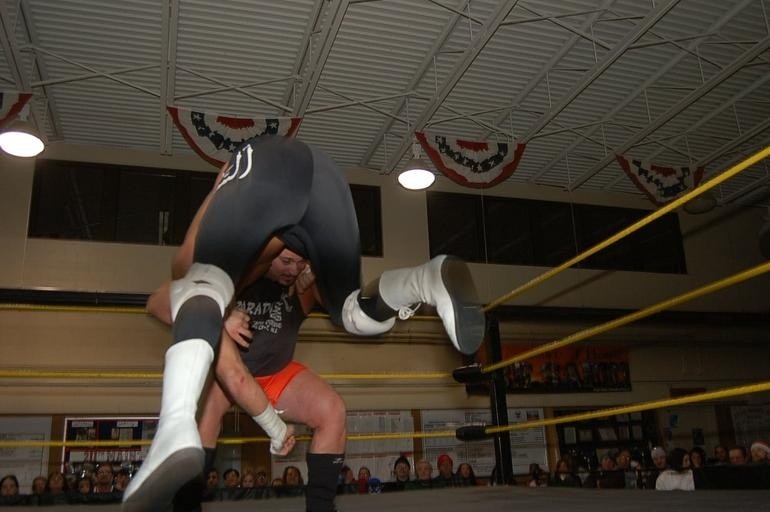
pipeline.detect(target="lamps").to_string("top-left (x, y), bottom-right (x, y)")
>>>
top-left (0, 49), bottom-right (48, 160)
top-left (397, 139), bottom-right (436, 191)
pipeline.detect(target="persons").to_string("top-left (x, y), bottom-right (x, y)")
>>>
top-left (405, 458), bottom-right (446, 488)
top-left (45, 461), bottom-right (131, 494)
top-left (2, 475), bottom-right (19, 496)
top-left (689, 447), bottom-right (706, 466)
top-left (728, 445), bottom-right (749, 465)
top-left (655, 448), bottom-right (696, 491)
top-left (529, 469), bottom-right (548, 488)
top-left (255, 471), bottom-right (265, 488)
top-left (553, 443), bottom-right (669, 490)
top-left (452, 463), bottom-right (476, 486)
top-left (487, 465), bottom-right (497, 487)
top-left (381, 456), bottom-right (417, 493)
top-left (33, 476), bottom-right (48, 495)
top-left (750, 439), bottom-right (768, 464)
top-left (283, 466), bottom-right (303, 486)
top-left (272, 479), bottom-right (284, 487)
top-left (712, 444), bottom-right (729, 465)
top-left (206, 467), bottom-right (219, 488)
top-left (122, 137), bottom-right (486, 509)
top-left (223, 469), bottom-right (240, 488)
top-left (145, 248), bottom-right (347, 509)
top-left (241, 469), bottom-right (257, 488)
top-left (353, 467), bottom-right (370, 494)
top-left (433, 453), bottom-right (462, 487)
top-left (339, 466), bottom-right (360, 494)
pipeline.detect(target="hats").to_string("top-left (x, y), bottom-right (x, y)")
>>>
top-left (750, 439), bottom-right (770, 454)
top-left (394, 454), bottom-right (412, 472)
top-left (437, 454), bottom-right (454, 468)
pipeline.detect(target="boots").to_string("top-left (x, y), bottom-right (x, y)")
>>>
top-left (377, 251), bottom-right (487, 357)
top-left (117, 336), bottom-right (218, 512)
